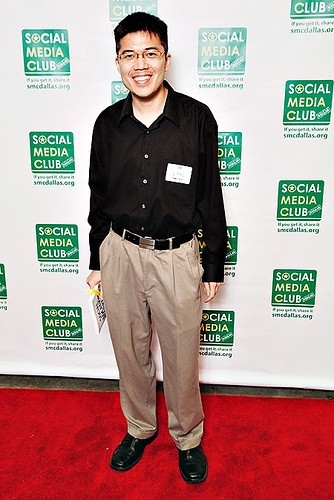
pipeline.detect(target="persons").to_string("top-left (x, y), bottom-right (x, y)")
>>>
top-left (85, 13), bottom-right (228, 486)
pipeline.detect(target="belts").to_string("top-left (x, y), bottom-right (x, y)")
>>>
top-left (112, 221), bottom-right (194, 250)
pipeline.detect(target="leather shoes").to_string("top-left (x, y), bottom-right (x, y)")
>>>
top-left (110, 430), bottom-right (158, 471)
top-left (179, 445), bottom-right (207, 483)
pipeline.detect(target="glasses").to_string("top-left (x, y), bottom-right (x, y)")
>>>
top-left (115, 51), bottom-right (164, 61)
